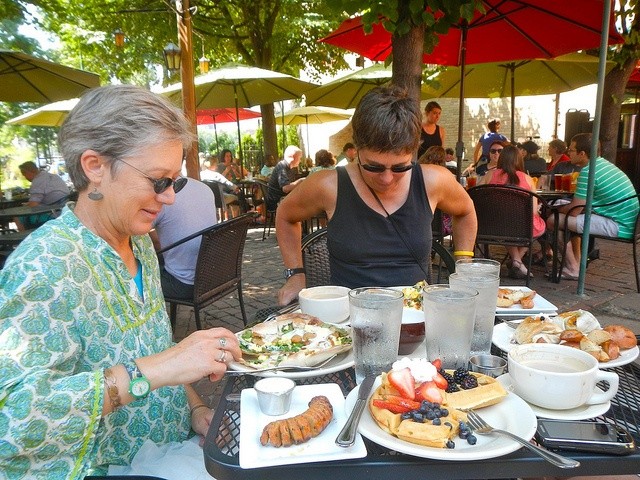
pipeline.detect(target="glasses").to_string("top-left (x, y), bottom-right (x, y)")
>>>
top-left (357, 150), bottom-right (416, 172)
top-left (490, 149), bottom-right (502, 154)
top-left (566, 149), bottom-right (580, 152)
top-left (115, 156), bottom-right (188, 193)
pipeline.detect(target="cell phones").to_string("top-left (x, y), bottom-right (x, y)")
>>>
top-left (534, 421), bottom-right (637, 457)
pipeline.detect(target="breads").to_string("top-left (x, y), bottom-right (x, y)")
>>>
top-left (368, 356), bottom-right (510, 447)
top-left (497, 288), bottom-right (537, 309)
top-left (513, 308), bottom-right (638, 363)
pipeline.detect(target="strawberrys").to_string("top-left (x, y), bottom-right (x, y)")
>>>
top-left (373, 397), bottom-right (410, 411)
top-left (414, 380), bottom-right (442, 404)
top-left (388, 368), bottom-right (416, 403)
top-left (433, 373), bottom-right (447, 388)
top-left (432, 359), bottom-right (440, 368)
top-left (386, 395), bottom-right (419, 408)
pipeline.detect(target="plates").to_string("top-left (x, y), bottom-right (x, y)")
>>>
top-left (495, 372), bottom-right (612, 421)
top-left (346, 374), bottom-right (538, 462)
top-left (237, 381), bottom-right (368, 470)
top-left (386, 286), bottom-right (424, 294)
top-left (225, 320), bottom-right (356, 379)
top-left (496, 286), bottom-right (559, 321)
top-left (492, 318), bottom-right (640, 369)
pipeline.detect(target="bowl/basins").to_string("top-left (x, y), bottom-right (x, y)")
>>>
top-left (351, 307), bottom-right (426, 355)
top-left (298, 285), bottom-right (352, 324)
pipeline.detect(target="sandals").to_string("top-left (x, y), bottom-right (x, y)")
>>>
top-left (541, 253), bottom-right (557, 268)
top-left (506, 260), bottom-right (534, 279)
top-left (528, 250), bottom-right (543, 264)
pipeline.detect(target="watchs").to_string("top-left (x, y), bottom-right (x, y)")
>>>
top-left (282, 267), bottom-right (306, 280)
top-left (122, 357), bottom-right (150, 402)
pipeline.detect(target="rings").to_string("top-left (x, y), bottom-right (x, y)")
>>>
top-left (218, 350), bottom-right (226, 362)
top-left (220, 338), bottom-right (226, 348)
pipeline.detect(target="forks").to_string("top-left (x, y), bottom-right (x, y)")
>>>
top-left (458, 407), bottom-right (581, 470)
top-left (225, 353), bottom-right (339, 374)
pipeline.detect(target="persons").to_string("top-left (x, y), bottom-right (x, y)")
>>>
top-left (418, 101), bottom-right (445, 159)
top-left (419, 146), bottom-right (454, 234)
top-left (0, 83), bottom-right (242, 479)
top-left (474, 118), bottom-right (512, 164)
top-left (13, 162), bottom-right (69, 232)
top-left (522, 141), bottom-right (546, 178)
top-left (529, 161), bottom-right (582, 264)
top-left (309, 149), bottom-right (335, 175)
top-left (445, 148), bottom-right (457, 167)
top-left (335, 143), bottom-right (359, 166)
top-left (260, 155), bottom-right (276, 180)
top-left (152, 175), bottom-right (218, 299)
top-left (199, 156), bottom-right (240, 222)
top-left (265, 145), bottom-right (306, 212)
top-left (273, 86), bottom-right (479, 306)
top-left (475, 140), bottom-right (508, 178)
top-left (545, 140), bottom-right (572, 172)
top-left (477, 145), bottom-right (545, 280)
top-left (217, 149), bottom-right (242, 222)
top-left (544, 132), bottom-right (639, 280)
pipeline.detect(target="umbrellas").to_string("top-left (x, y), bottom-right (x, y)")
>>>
top-left (315, 0), bottom-right (626, 185)
top-left (273, 105), bottom-right (353, 156)
top-left (301, 60), bottom-right (442, 110)
top-left (195, 109), bottom-right (264, 152)
top-left (416, 51), bottom-right (618, 146)
top-left (153, 57), bottom-right (322, 160)
top-left (4, 97), bottom-right (87, 127)
top-left (1, 50), bottom-right (103, 105)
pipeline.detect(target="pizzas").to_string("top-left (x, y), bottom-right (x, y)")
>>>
top-left (237, 312), bottom-right (352, 371)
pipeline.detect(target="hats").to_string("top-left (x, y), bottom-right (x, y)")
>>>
top-left (522, 140), bottom-right (538, 153)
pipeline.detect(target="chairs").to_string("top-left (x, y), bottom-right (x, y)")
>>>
top-left (155, 211), bottom-right (255, 335)
top-left (0, 228), bottom-right (18, 258)
top-left (466, 183), bottom-right (560, 288)
top-left (311, 211), bottom-right (327, 233)
top-left (203, 180), bottom-right (242, 221)
top-left (559, 194), bottom-right (640, 295)
top-left (431, 207), bottom-right (452, 259)
top-left (447, 165), bottom-right (456, 175)
top-left (254, 178), bottom-right (288, 241)
top-left (300, 226), bottom-right (455, 288)
top-left (23, 191), bottom-right (79, 230)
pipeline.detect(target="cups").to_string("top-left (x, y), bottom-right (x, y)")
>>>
top-left (541, 175), bottom-right (552, 192)
top-left (561, 173), bottom-right (573, 193)
top-left (454, 258), bottom-right (501, 277)
top-left (554, 174), bottom-right (563, 193)
top-left (422, 283), bottom-right (479, 371)
top-left (467, 176), bottom-right (478, 189)
top-left (507, 342), bottom-right (619, 411)
top-left (348, 286), bottom-right (405, 387)
top-left (469, 354), bottom-right (508, 378)
top-left (246, 171), bottom-right (253, 181)
top-left (448, 272), bottom-right (501, 371)
top-left (4, 189), bottom-right (13, 200)
top-left (253, 376), bottom-right (297, 417)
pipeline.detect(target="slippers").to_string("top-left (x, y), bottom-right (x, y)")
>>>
top-left (545, 271), bottom-right (579, 281)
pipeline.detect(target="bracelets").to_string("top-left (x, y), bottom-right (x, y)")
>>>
top-left (191, 404), bottom-right (210, 415)
top-left (453, 250), bottom-right (474, 257)
top-left (103, 368), bottom-right (121, 410)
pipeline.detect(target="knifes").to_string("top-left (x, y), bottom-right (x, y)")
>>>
top-left (335, 373), bottom-right (376, 448)
